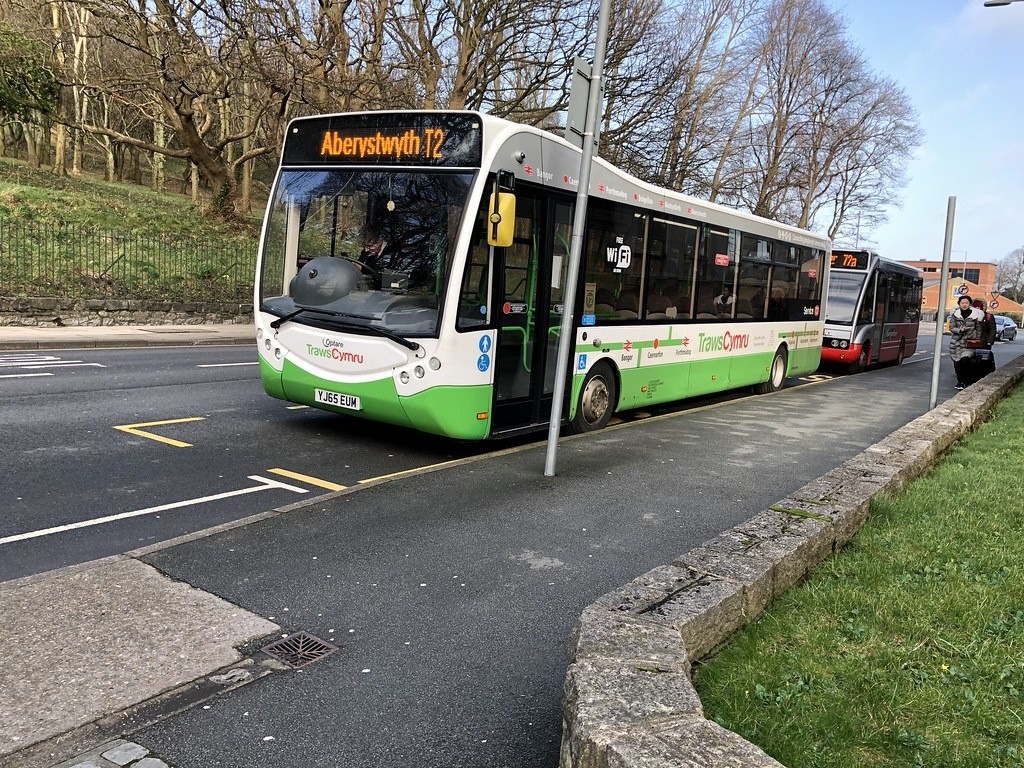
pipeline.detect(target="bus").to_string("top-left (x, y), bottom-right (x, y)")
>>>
top-left (249, 108), bottom-right (834, 447)
top-left (821, 249), bottom-right (925, 371)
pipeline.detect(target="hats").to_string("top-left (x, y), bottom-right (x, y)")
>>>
top-left (974, 301), bottom-right (982, 308)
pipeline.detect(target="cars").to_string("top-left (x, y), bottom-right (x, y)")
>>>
top-left (992, 314), bottom-right (1018, 342)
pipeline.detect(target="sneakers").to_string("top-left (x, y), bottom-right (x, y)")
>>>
top-left (954, 382), bottom-right (965, 390)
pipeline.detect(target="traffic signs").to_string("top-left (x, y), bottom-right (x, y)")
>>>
top-left (990, 300), bottom-right (999, 308)
top-left (959, 285), bottom-right (969, 295)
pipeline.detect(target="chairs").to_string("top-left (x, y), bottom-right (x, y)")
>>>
top-left (473, 267), bottom-right (783, 318)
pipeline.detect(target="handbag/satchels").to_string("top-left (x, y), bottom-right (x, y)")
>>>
top-left (964, 338), bottom-right (983, 348)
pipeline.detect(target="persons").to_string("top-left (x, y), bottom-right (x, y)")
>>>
top-left (970, 297), bottom-right (996, 349)
top-left (346, 223), bottom-right (421, 292)
top-left (712, 286), bottom-right (737, 317)
top-left (948, 296), bottom-right (985, 390)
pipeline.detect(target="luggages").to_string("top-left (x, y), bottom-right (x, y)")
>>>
top-left (969, 349), bottom-right (995, 382)
